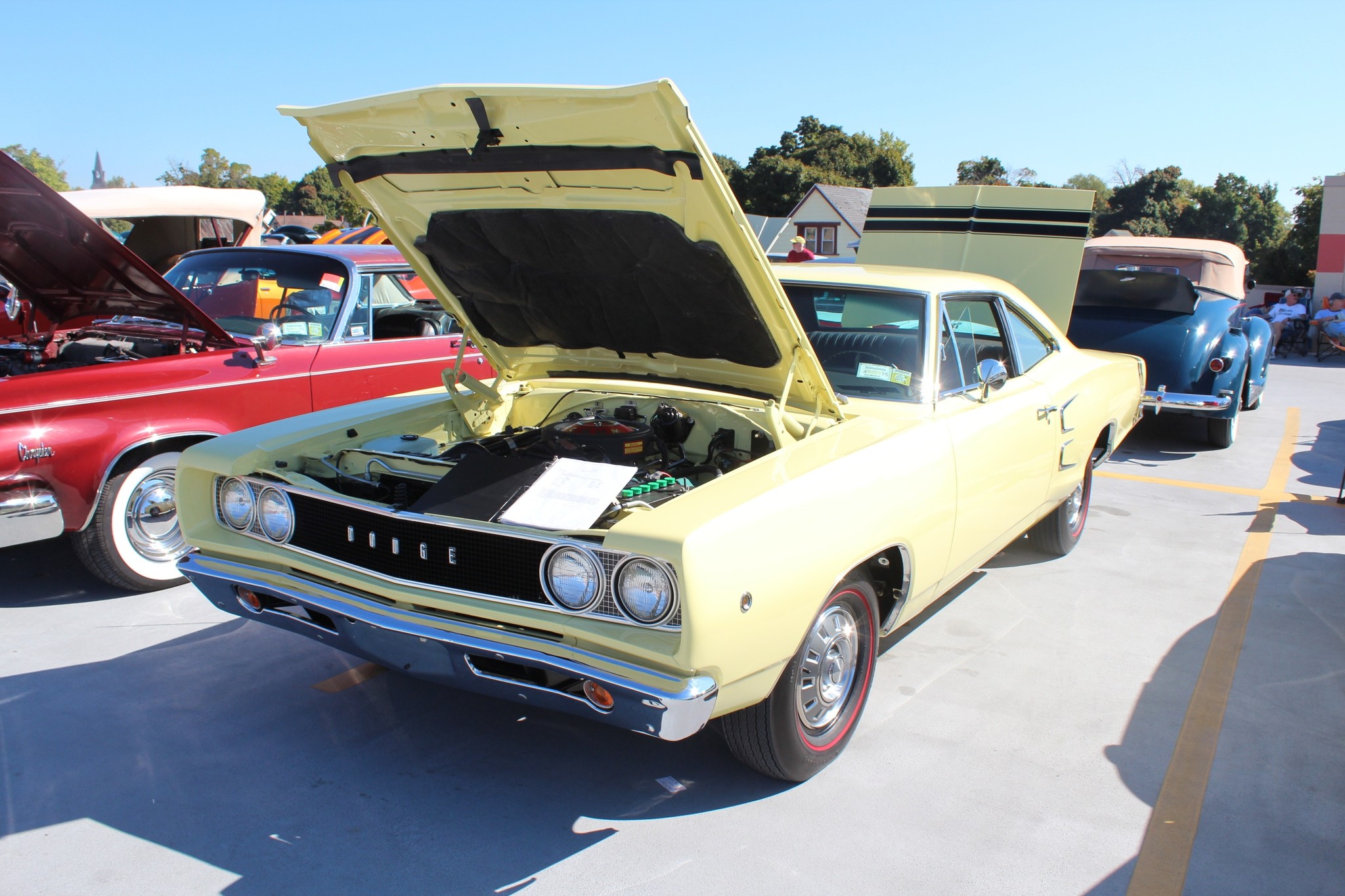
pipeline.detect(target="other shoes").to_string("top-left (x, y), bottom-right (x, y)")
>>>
top-left (1269, 352), bottom-right (1276, 359)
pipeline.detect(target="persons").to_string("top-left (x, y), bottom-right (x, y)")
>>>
top-left (1314, 292), bottom-right (1345, 348)
top-left (786, 235), bottom-right (815, 263)
top-left (1249, 288), bottom-right (1307, 360)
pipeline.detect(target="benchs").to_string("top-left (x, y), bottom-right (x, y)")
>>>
top-left (348, 310), bottom-right (449, 337)
top-left (801, 328), bottom-right (1001, 392)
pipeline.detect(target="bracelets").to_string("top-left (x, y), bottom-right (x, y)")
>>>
top-left (1287, 317), bottom-right (1290, 322)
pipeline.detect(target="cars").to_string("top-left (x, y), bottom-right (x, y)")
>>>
top-left (1065, 235), bottom-right (1274, 451)
top-left (166, 76), bottom-right (1148, 787)
top-left (0, 150), bottom-right (499, 596)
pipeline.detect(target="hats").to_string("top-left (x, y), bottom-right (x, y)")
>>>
top-left (1284, 288), bottom-right (1303, 299)
top-left (790, 236), bottom-right (806, 244)
top-left (1327, 292), bottom-right (1345, 301)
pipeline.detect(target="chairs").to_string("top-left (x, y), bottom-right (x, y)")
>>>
top-left (202, 237), bottom-right (228, 249)
top-left (285, 288), bottom-right (334, 318)
top-left (372, 312), bottom-right (439, 341)
top-left (1248, 291), bottom-right (1345, 363)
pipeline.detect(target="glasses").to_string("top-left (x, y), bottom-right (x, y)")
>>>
top-left (1293, 294), bottom-right (1300, 297)
